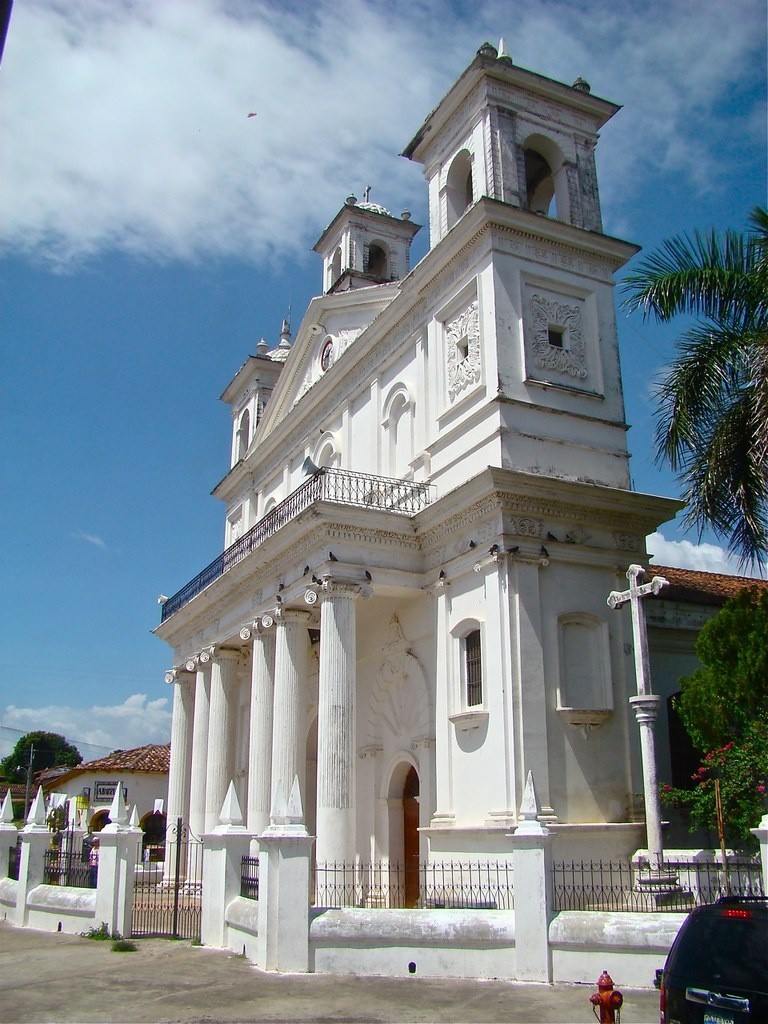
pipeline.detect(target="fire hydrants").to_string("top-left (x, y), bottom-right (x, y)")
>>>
top-left (589, 970), bottom-right (623, 1024)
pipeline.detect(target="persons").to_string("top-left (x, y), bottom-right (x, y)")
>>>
top-left (89, 837), bottom-right (99, 888)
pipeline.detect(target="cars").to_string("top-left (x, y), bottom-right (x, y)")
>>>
top-left (654, 894), bottom-right (768, 1024)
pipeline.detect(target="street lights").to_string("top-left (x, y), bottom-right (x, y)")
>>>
top-left (17, 766), bottom-right (31, 826)
